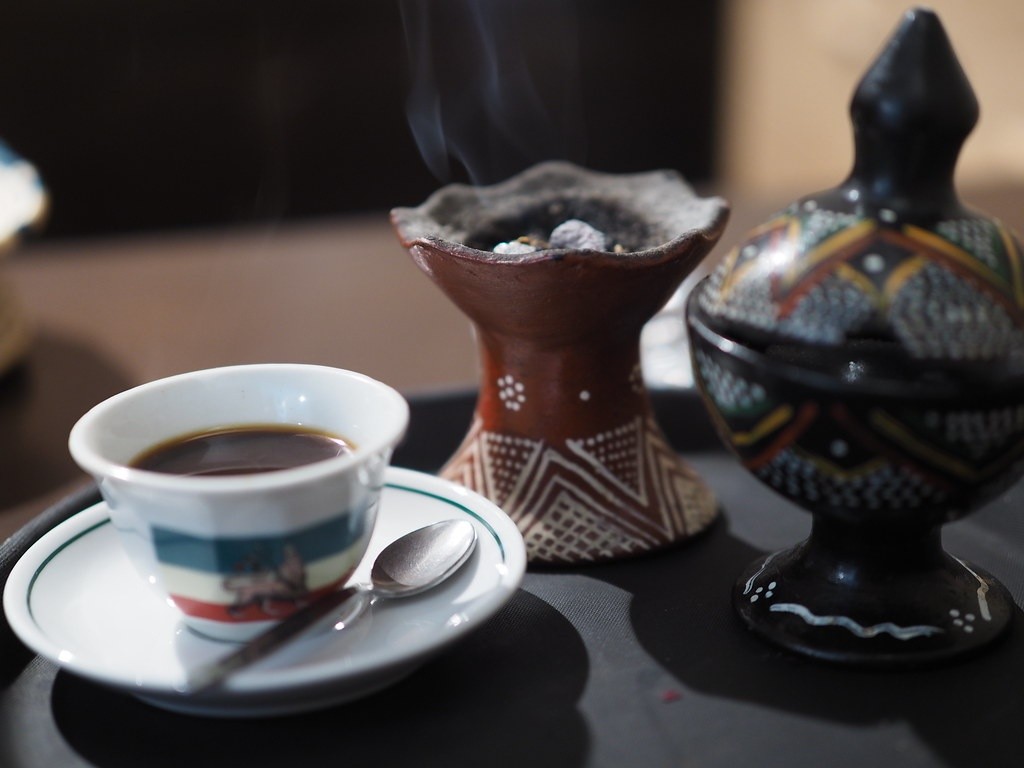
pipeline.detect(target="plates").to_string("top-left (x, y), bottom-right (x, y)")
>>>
top-left (2, 466), bottom-right (527, 720)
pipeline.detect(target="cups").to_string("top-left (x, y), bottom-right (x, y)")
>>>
top-left (69, 365), bottom-right (410, 648)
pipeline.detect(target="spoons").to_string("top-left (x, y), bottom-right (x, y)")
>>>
top-left (172, 518), bottom-right (479, 695)
